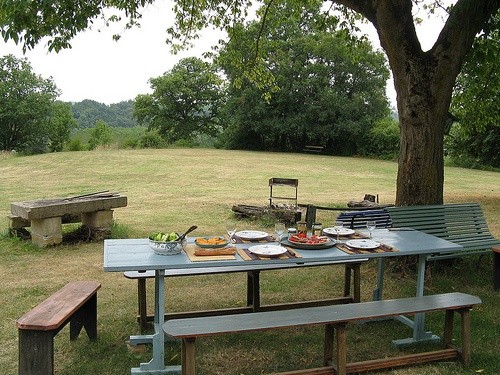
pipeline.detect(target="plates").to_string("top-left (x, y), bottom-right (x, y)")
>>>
top-left (347, 239), bottom-right (381, 249)
top-left (248, 245), bottom-right (287, 256)
top-left (235, 230), bottom-right (269, 240)
top-left (287, 236), bottom-right (331, 244)
top-left (195, 237), bottom-right (230, 248)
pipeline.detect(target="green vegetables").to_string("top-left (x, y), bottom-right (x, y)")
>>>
top-left (148, 232), bottom-right (178, 242)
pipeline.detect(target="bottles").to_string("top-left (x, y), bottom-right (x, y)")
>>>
top-left (323, 227), bottom-right (356, 236)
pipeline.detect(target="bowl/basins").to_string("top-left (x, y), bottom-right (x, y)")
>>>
top-left (148, 234), bottom-right (188, 255)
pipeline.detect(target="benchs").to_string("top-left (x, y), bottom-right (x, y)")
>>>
top-left (17, 279), bottom-right (101, 375)
top-left (7, 213), bottom-right (82, 240)
top-left (384, 202), bottom-right (500, 277)
top-left (162, 292), bottom-right (482, 375)
top-left (303, 145), bottom-right (323, 154)
top-left (123, 262), bottom-right (361, 333)
top-left (298, 203), bottom-right (395, 229)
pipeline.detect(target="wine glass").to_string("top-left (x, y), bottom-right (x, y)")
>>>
top-left (274, 223), bottom-right (286, 246)
top-left (225, 222), bottom-right (237, 244)
top-left (335, 222), bottom-right (343, 244)
top-left (367, 220), bottom-right (376, 239)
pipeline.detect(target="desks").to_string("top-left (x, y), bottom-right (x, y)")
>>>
top-left (11, 192), bottom-right (127, 248)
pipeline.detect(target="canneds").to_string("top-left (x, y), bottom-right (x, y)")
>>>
top-left (288, 228), bottom-right (296, 240)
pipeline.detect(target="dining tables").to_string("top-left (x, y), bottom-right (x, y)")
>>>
top-left (103, 227), bottom-right (463, 375)
